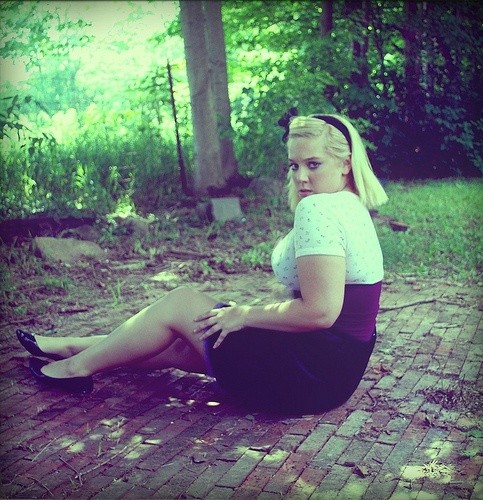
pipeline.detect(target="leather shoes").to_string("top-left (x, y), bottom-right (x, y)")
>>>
top-left (16, 329), bottom-right (64, 360)
top-left (29, 356), bottom-right (94, 394)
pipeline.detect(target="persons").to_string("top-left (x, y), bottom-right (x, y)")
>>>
top-left (15, 113), bottom-right (389, 416)
